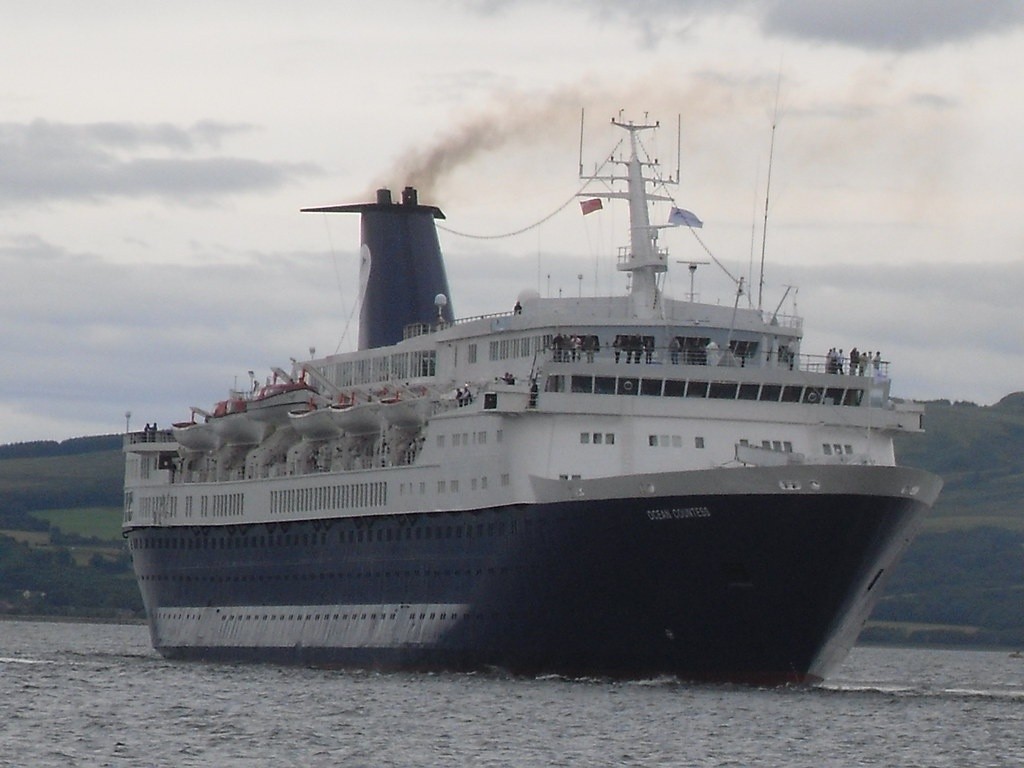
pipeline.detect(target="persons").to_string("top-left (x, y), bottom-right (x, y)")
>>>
top-left (332, 437), bottom-right (416, 472)
top-left (455, 372), bottom-right (538, 409)
top-left (548, 333), bottom-right (881, 376)
top-left (514, 301), bottom-right (523, 314)
top-left (143, 422), bottom-right (157, 442)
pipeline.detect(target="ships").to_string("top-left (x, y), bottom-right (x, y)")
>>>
top-left (121, 108), bottom-right (945, 691)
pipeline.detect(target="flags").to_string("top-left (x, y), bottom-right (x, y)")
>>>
top-left (581, 198), bottom-right (603, 215)
top-left (668, 207), bottom-right (703, 228)
